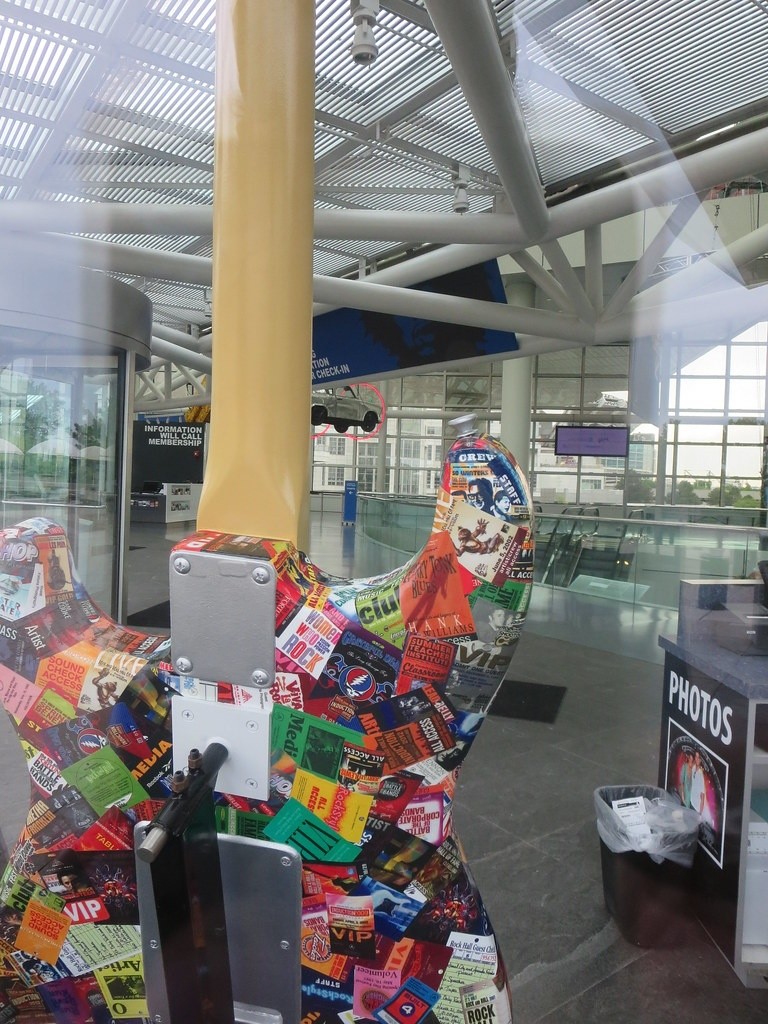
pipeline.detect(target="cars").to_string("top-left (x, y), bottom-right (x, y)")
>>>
top-left (311, 385), bottom-right (385, 434)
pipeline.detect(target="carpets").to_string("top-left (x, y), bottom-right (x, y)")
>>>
top-left (486, 680), bottom-right (569, 725)
top-left (127, 601), bottom-right (171, 629)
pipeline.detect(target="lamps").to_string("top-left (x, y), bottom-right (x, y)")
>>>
top-left (205, 303), bottom-right (212, 317)
top-left (351, 19), bottom-right (379, 65)
top-left (452, 188), bottom-right (469, 213)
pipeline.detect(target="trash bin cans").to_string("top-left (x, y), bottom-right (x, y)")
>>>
top-left (592, 784), bottom-right (699, 950)
